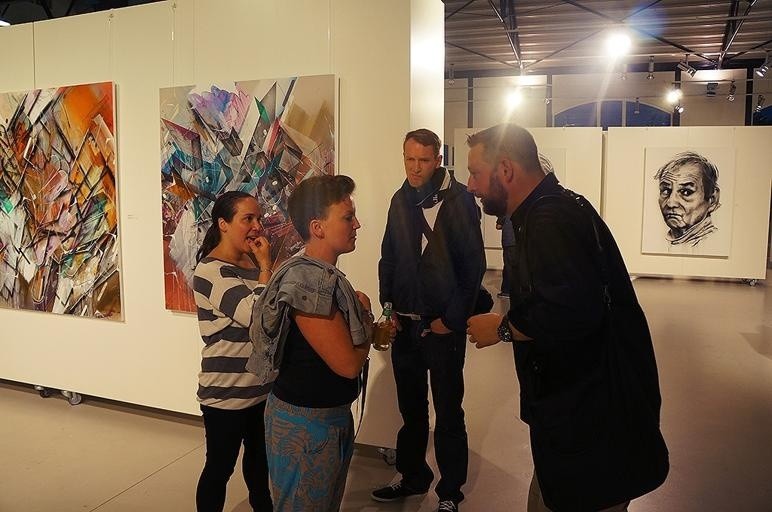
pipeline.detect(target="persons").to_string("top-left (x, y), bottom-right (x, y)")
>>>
top-left (655, 150), bottom-right (721, 246)
top-left (250, 174), bottom-right (396, 512)
top-left (191, 191), bottom-right (274, 512)
top-left (370, 129), bottom-right (486, 511)
top-left (464, 123), bottom-right (670, 511)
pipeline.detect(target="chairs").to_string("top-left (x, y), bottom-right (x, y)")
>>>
top-left (370, 473), bottom-right (432, 503)
top-left (434, 499), bottom-right (460, 512)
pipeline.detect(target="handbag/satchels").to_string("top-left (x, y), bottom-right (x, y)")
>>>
top-left (258, 268), bottom-right (274, 274)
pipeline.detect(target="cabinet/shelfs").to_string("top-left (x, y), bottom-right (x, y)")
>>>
top-left (374, 301), bottom-right (395, 352)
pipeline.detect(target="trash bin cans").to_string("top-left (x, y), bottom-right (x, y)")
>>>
top-left (497, 316), bottom-right (513, 343)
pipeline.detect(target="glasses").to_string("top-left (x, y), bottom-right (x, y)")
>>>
top-left (618, 51), bottom-right (771, 117)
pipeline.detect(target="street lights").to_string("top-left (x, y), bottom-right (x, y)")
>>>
top-left (468, 282), bottom-right (496, 316)
top-left (517, 195), bottom-right (669, 512)
